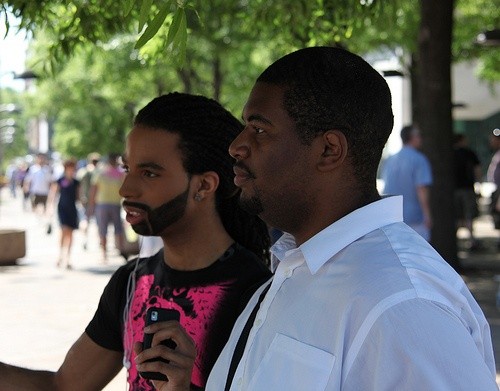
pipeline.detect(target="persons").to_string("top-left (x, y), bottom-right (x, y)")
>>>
top-left (206, 46), bottom-right (500, 391)
top-left (487, 129), bottom-right (500, 234)
top-left (381, 123), bottom-right (432, 244)
top-left (450, 134), bottom-right (483, 242)
top-left (9, 152), bottom-right (134, 271)
top-left (0, 92), bottom-right (271, 390)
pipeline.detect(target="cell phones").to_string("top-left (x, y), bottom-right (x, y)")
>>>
top-left (139, 307), bottom-right (182, 382)
